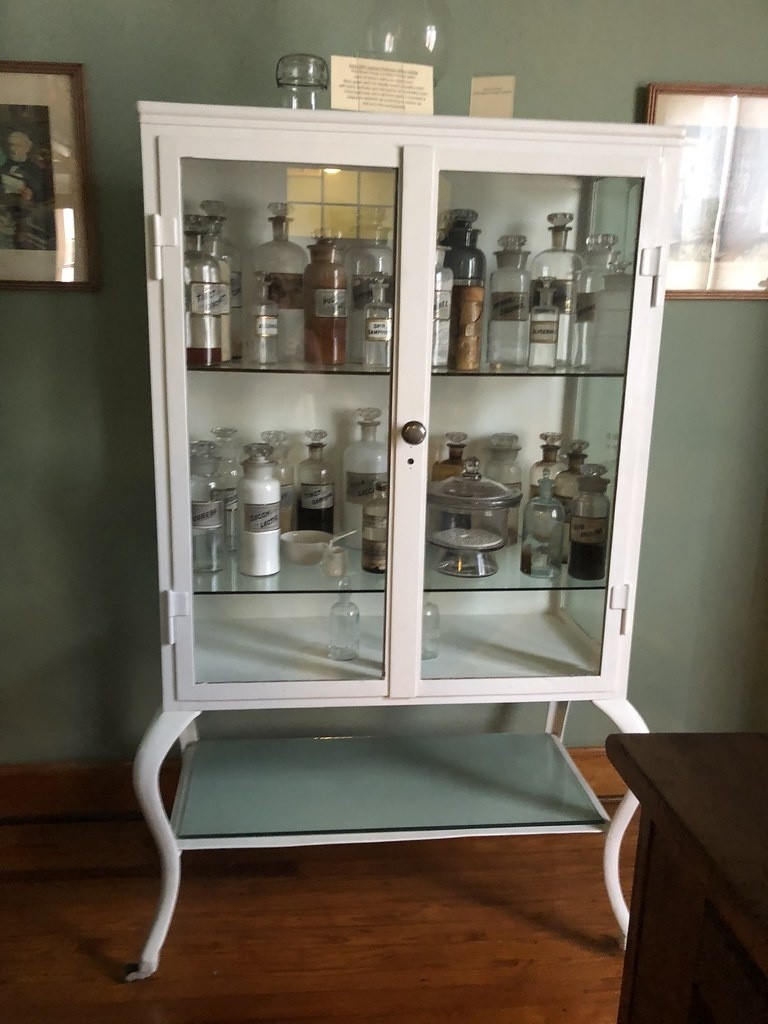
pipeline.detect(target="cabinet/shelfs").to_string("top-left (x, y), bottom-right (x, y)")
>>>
top-left (123, 95), bottom-right (692, 985)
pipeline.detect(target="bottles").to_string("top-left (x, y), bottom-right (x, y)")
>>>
top-left (432, 209), bottom-right (633, 373)
top-left (184, 200), bottom-right (392, 370)
top-left (422, 593), bottom-right (439, 661)
top-left (330, 580), bottom-right (360, 659)
top-left (190, 407), bottom-right (388, 575)
top-left (431, 431), bottom-right (613, 580)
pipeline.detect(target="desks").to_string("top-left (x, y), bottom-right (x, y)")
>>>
top-left (605, 732), bottom-right (768, 1024)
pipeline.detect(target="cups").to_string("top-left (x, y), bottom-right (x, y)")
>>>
top-left (324, 547), bottom-right (346, 576)
top-left (276, 54), bottom-right (329, 110)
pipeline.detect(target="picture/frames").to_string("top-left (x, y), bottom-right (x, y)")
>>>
top-left (0, 60), bottom-right (106, 294)
top-left (644, 83), bottom-right (768, 301)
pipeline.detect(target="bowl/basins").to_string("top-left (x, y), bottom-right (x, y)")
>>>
top-left (281, 527), bottom-right (358, 567)
